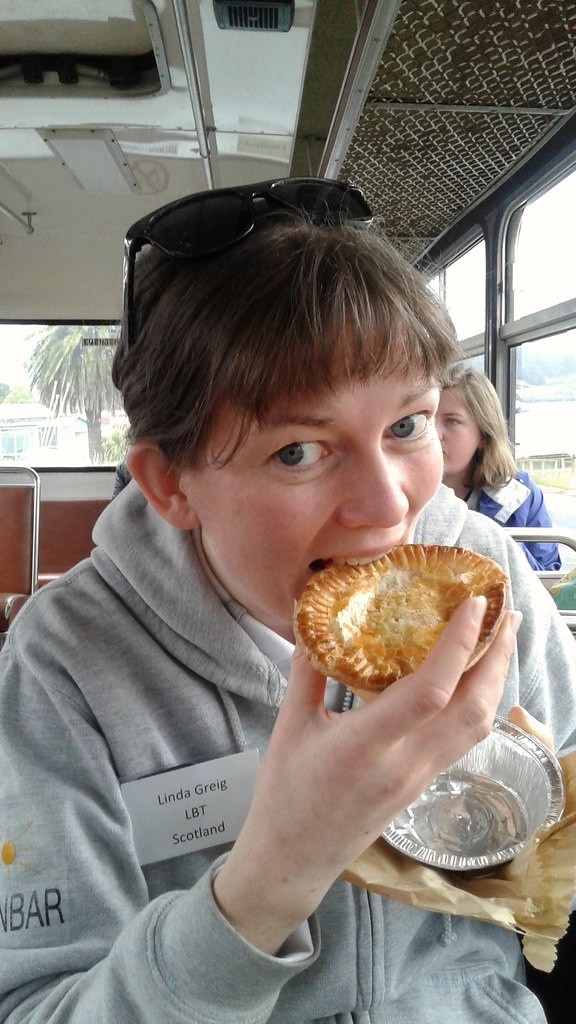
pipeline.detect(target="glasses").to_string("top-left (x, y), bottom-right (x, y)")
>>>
top-left (123, 178), bottom-right (374, 362)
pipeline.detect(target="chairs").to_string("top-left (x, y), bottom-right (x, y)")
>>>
top-left (0, 461), bottom-right (121, 645)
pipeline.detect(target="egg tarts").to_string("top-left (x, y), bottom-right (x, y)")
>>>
top-left (294, 545), bottom-right (508, 696)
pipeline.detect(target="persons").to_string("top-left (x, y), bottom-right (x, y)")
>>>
top-left (0, 180), bottom-right (576, 1017)
top-left (435, 364), bottom-right (562, 572)
